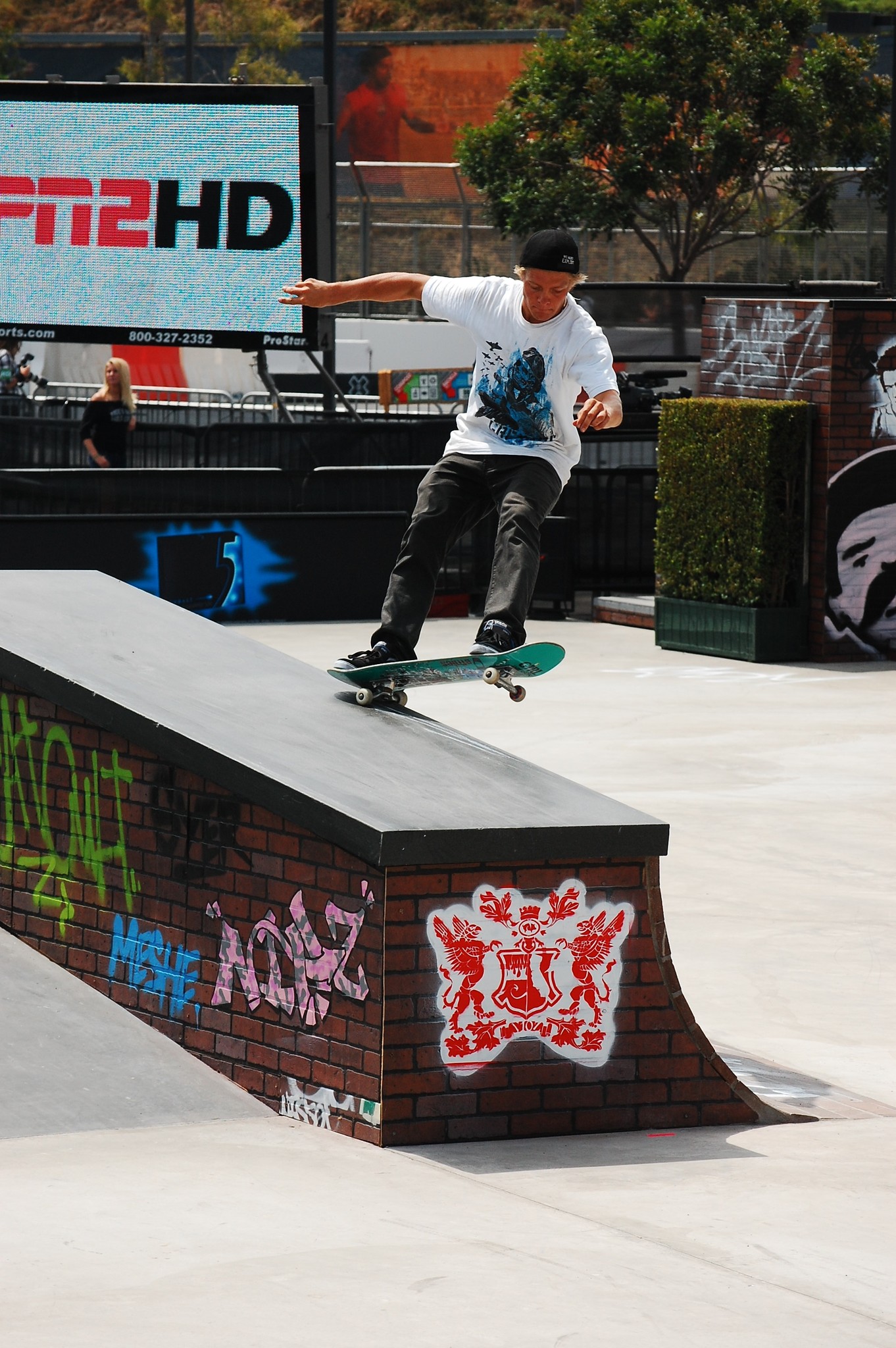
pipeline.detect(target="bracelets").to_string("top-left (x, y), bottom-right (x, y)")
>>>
top-left (93, 454), bottom-right (99, 460)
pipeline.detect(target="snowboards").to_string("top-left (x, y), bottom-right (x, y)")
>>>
top-left (326, 639), bottom-right (565, 707)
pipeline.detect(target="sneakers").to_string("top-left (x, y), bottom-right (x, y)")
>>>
top-left (333, 635), bottom-right (401, 669)
top-left (469, 618), bottom-right (515, 654)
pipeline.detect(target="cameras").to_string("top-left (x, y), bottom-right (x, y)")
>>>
top-left (14, 354), bottom-right (47, 389)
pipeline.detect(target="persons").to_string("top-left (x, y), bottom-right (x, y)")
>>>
top-left (80, 358), bottom-right (137, 468)
top-left (0, 339), bottom-right (31, 395)
top-left (276, 231), bottom-right (622, 669)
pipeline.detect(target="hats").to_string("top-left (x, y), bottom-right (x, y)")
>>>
top-left (520, 229), bottom-right (580, 274)
